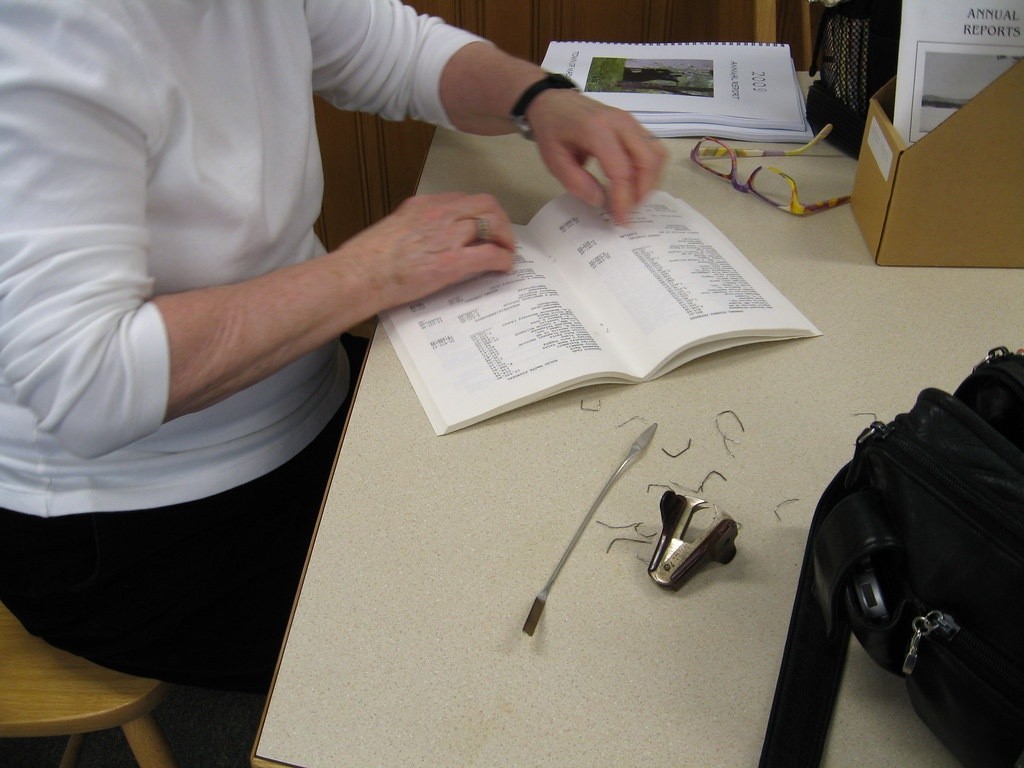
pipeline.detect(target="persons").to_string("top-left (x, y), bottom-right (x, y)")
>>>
top-left (0, 0), bottom-right (670, 686)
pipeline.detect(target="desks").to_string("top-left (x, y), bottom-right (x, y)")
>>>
top-left (246, 70), bottom-right (1023, 768)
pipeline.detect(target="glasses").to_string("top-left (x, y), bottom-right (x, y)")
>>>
top-left (689, 125), bottom-right (852, 216)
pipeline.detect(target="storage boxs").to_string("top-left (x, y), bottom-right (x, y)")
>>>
top-left (849, 55), bottom-right (1024, 268)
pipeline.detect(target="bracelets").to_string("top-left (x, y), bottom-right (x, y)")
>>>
top-left (509, 73), bottom-right (576, 133)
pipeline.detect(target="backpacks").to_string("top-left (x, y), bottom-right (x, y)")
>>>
top-left (757, 346), bottom-right (1023, 767)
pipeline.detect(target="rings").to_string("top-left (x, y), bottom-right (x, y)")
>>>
top-left (477, 220), bottom-right (490, 239)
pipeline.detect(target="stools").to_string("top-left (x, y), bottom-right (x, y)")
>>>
top-left (0, 603), bottom-right (180, 768)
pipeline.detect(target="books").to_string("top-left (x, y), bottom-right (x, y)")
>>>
top-left (353, 188), bottom-right (825, 438)
top-left (540, 43), bottom-right (815, 143)
